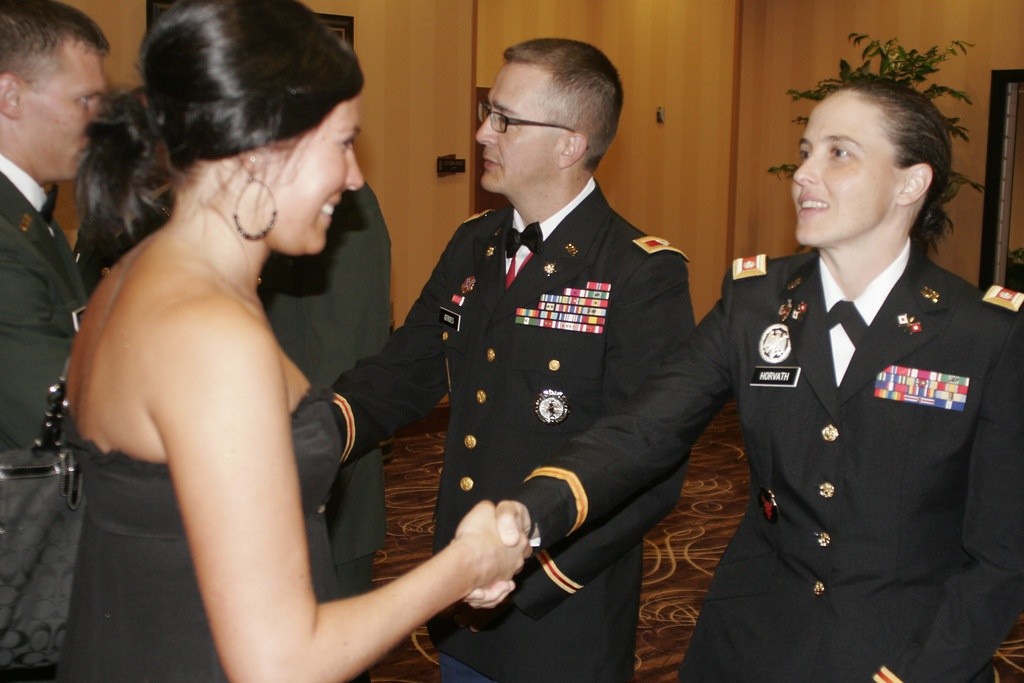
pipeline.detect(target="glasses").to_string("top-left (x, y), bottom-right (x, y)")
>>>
top-left (477, 101), bottom-right (576, 134)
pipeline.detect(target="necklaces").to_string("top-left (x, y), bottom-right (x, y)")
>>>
top-left (157, 202), bottom-right (262, 290)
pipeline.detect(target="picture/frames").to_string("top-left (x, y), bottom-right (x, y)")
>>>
top-left (314, 12), bottom-right (354, 51)
top-left (147, 0), bottom-right (174, 32)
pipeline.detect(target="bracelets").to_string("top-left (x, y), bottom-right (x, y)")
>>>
top-left (521, 504), bottom-right (535, 539)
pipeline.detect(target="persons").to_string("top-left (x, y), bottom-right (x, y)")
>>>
top-left (59, 1), bottom-right (533, 683)
top-left (257, 182), bottom-right (394, 683)
top-left (1, 0), bottom-right (113, 456)
top-left (454, 69), bottom-right (1024, 682)
top-left (327, 37), bottom-right (697, 682)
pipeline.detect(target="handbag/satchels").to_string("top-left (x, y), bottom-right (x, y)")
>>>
top-left (0, 381), bottom-right (84, 682)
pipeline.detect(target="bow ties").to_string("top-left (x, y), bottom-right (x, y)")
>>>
top-left (504, 221), bottom-right (543, 259)
top-left (40, 183), bottom-right (58, 226)
